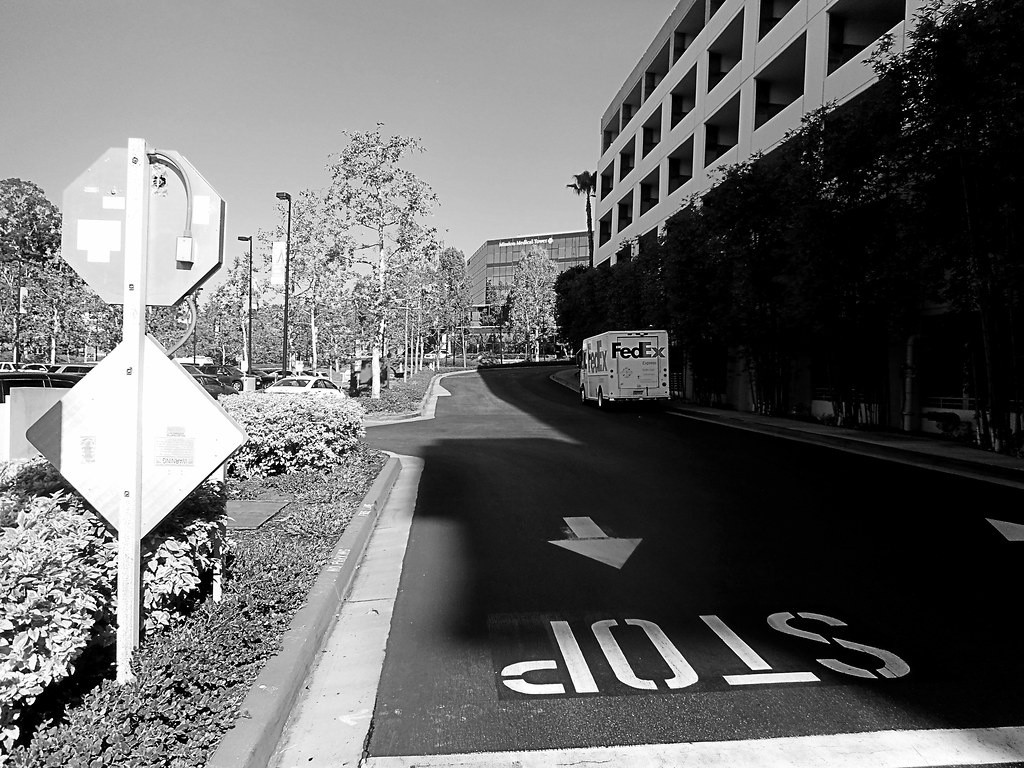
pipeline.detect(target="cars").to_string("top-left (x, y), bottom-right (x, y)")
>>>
top-left (0, 360), bottom-right (352, 405)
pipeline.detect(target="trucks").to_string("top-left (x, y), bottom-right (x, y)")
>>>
top-left (575, 330), bottom-right (669, 408)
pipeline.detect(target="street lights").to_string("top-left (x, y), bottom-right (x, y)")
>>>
top-left (238, 236), bottom-right (253, 378)
top-left (275, 192), bottom-right (292, 380)
